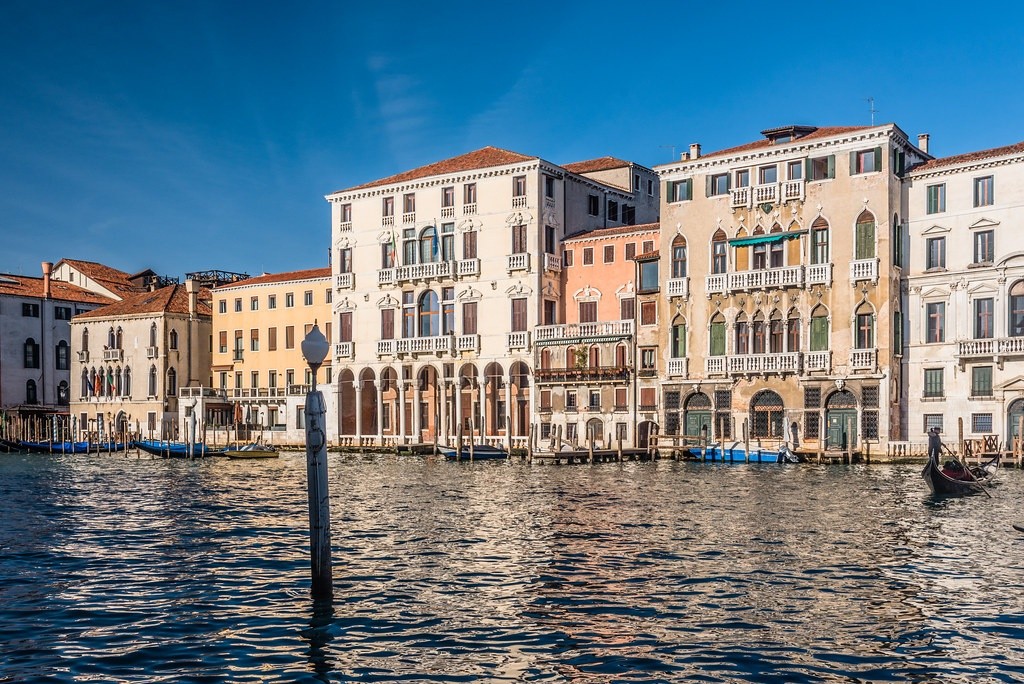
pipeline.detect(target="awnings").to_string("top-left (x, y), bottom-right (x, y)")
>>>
top-left (728, 229), bottom-right (809, 247)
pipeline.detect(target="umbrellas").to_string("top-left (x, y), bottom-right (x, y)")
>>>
top-left (233, 401), bottom-right (242, 451)
top-left (245, 403), bottom-right (251, 445)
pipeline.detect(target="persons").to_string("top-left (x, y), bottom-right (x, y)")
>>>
top-left (928, 425), bottom-right (946, 467)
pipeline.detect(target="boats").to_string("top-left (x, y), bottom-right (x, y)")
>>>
top-left (131, 440), bottom-right (209, 459)
top-left (22, 439), bottom-right (137, 452)
top-left (438, 444), bottom-right (508, 459)
top-left (221, 440), bottom-right (280, 457)
top-left (688, 444), bottom-right (800, 464)
top-left (920, 439), bottom-right (1003, 497)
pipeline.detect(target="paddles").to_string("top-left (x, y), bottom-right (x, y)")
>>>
top-left (941, 441), bottom-right (991, 499)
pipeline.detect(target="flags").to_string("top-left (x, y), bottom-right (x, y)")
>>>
top-left (389, 230), bottom-right (396, 264)
top-left (94, 374), bottom-right (101, 394)
top-left (432, 221), bottom-right (439, 257)
top-left (105, 373), bottom-right (116, 390)
top-left (87, 377), bottom-right (93, 391)
